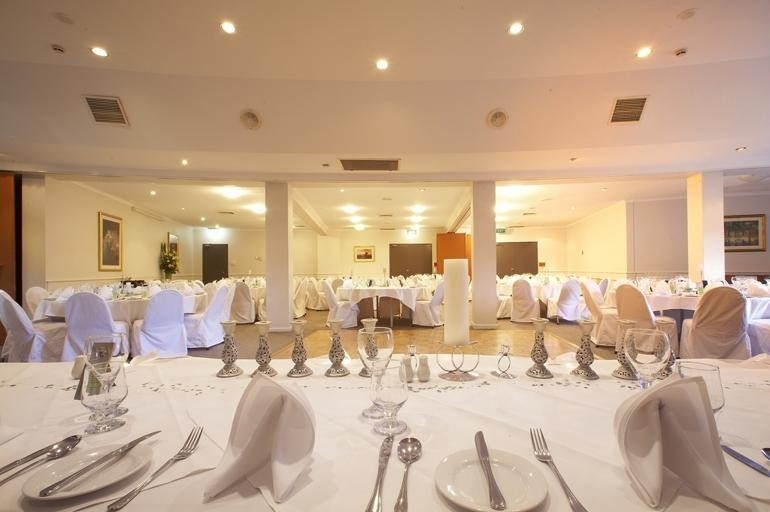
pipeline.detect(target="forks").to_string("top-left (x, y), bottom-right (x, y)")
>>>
top-left (107, 425), bottom-right (204, 511)
top-left (530, 427), bottom-right (588, 512)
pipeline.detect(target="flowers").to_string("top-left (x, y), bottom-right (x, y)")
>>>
top-left (160, 242), bottom-right (179, 274)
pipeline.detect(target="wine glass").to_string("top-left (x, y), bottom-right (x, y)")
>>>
top-left (81, 361), bottom-right (129, 434)
top-left (369, 359), bottom-right (409, 435)
top-left (83, 333), bottom-right (130, 422)
top-left (674, 360), bottom-right (725, 416)
top-left (357, 326), bottom-right (394, 419)
top-left (623, 328), bottom-right (671, 392)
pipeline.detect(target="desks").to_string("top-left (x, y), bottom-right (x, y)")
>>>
top-left (0, 355), bottom-right (770, 512)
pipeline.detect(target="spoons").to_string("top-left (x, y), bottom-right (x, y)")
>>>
top-left (0, 435), bottom-right (83, 487)
top-left (394, 437), bottom-right (421, 512)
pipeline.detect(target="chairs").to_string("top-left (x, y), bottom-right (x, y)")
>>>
top-left (675, 284), bottom-right (754, 360)
top-left (3, 274), bottom-right (769, 328)
top-left (579, 283), bottom-right (619, 349)
top-left (612, 285), bottom-right (676, 359)
top-left (178, 284), bottom-right (231, 351)
top-left (57, 289), bottom-right (129, 361)
top-left (746, 311), bottom-right (769, 352)
top-left (1, 289), bottom-right (65, 363)
top-left (130, 287), bottom-right (189, 359)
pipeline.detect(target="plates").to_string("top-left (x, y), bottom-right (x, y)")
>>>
top-left (434, 448), bottom-right (548, 512)
top-left (21, 444), bottom-right (153, 501)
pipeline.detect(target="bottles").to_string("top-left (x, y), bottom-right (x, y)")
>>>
top-left (417, 356), bottom-right (430, 382)
top-left (399, 358), bottom-right (413, 383)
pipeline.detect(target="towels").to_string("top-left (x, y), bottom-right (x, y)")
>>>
top-left (201, 370), bottom-right (318, 504)
top-left (612, 372), bottom-right (762, 511)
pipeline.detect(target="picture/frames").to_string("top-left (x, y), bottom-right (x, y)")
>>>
top-left (353, 245), bottom-right (374, 262)
top-left (725, 214), bottom-right (766, 251)
top-left (168, 233), bottom-right (179, 258)
top-left (98, 212), bottom-right (122, 271)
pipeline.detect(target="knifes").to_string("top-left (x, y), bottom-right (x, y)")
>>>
top-left (474, 431), bottom-right (507, 510)
top-left (720, 445), bottom-right (770, 479)
top-left (0, 441), bottom-right (60, 475)
top-left (39, 430), bottom-right (161, 497)
top-left (364, 436), bottom-right (393, 512)
